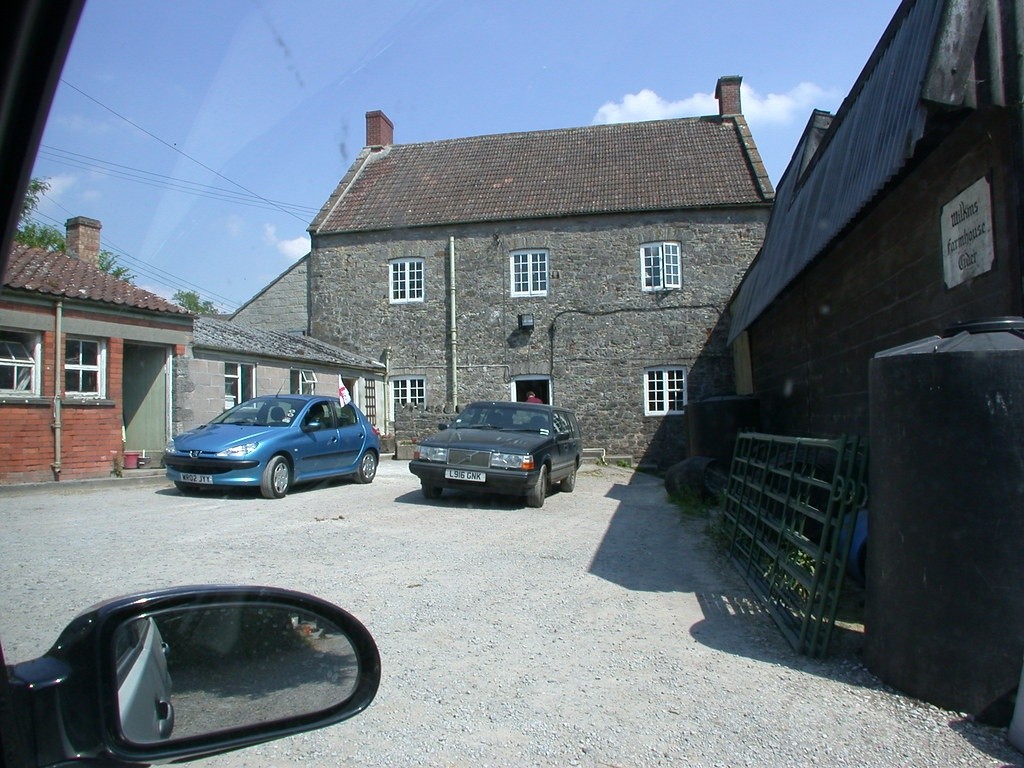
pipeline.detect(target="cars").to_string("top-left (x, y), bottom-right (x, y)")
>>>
top-left (413, 400), bottom-right (584, 508)
top-left (164, 396), bottom-right (381, 500)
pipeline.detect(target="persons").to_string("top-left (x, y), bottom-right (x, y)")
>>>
top-left (526, 391), bottom-right (543, 404)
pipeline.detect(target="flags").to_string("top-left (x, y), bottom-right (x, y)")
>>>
top-left (338, 374), bottom-right (351, 408)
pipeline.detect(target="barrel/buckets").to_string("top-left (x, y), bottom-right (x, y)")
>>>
top-left (138, 457), bottom-right (152, 469)
top-left (124, 452), bottom-right (141, 468)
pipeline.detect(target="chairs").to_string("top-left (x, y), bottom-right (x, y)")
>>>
top-left (304, 404), bottom-right (326, 430)
top-left (530, 416), bottom-right (545, 429)
top-left (485, 412), bottom-right (506, 425)
top-left (268, 407), bottom-right (288, 426)
